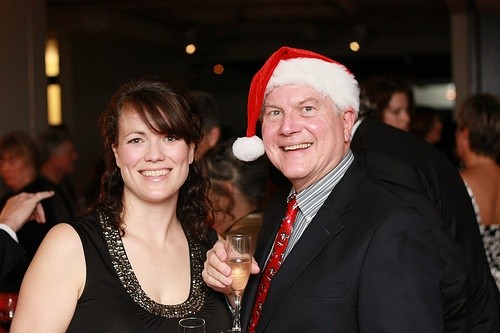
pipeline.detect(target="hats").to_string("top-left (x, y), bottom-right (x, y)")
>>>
top-left (232, 45), bottom-right (360, 161)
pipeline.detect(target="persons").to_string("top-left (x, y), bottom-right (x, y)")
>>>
top-left (350, 115), bottom-right (500, 332)
top-left (202, 44), bottom-right (446, 333)
top-left (9, 76), bottom-right (238, 332)
top-left (0, 63), bottom-right (465, 291)
top-left (454, 90), bottom-right (500, 289)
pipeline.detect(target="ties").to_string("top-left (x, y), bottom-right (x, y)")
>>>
top-left (248, 199), bottom-right (298, 333)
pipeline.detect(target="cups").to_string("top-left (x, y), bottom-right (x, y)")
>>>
top-left (179, 317), bottom-right (206, 333)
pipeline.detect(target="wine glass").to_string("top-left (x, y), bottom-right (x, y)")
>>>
top-left (224, 234), bottom-right (252, 333)
